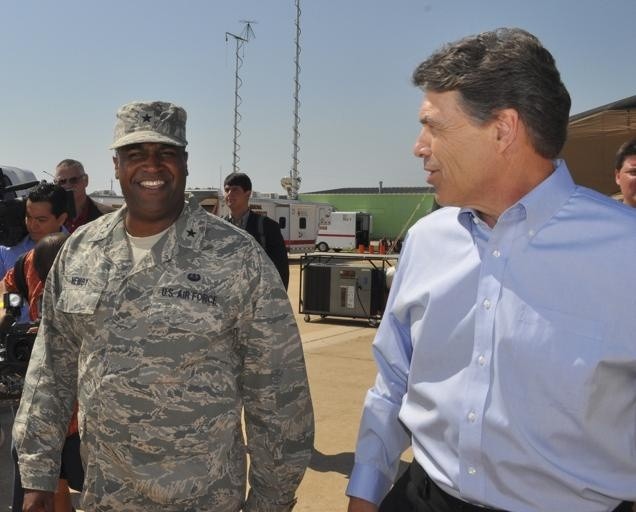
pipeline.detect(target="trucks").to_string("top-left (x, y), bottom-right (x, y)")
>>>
top-left (187, 190), bottom-right (373, 257)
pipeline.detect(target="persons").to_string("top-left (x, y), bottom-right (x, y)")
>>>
top-left (220, 171), bottom-right (290, 294)
top-left (12, 102), bottom-right (314, 511)
top-left (608, 137), bottom-right (636, 208)
top-left (347, 27), bottom-right (636, 512)
top-left (1, 159), bottom-right (123, 512)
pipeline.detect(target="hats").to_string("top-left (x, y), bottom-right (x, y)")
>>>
top-left (107, 98), bottom-right (188, 149)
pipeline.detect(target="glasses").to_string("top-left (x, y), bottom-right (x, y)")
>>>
top-left (56, 174), bottom-right (84, 185)
